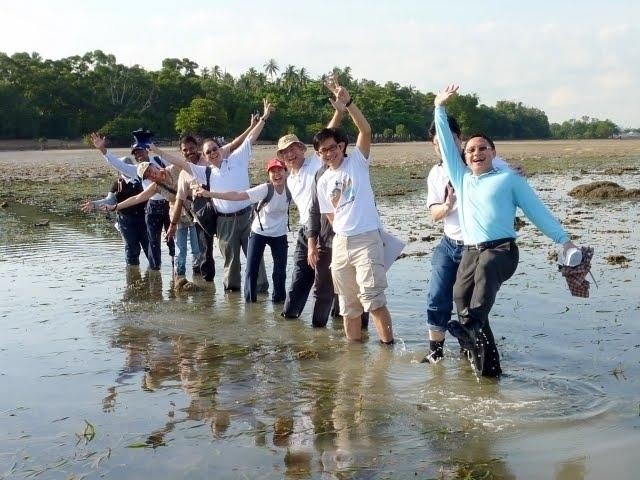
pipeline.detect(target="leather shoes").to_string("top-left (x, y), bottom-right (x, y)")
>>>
top-left (446, 318), bottom-right (482, 350)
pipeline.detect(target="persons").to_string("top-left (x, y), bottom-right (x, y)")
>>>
top-left (434, 85), bottom-right (578, 380)
top-left (148, 98), bottom-right (274, 293)
top-left (276, 133), bottom-right (353, 318)
top-left (307, 128), bottom-right (373, 330)
top-left (88, 132), bottom-right (171, 269)
top-left (313, 78), bottom-right (392, 344)
top-left (164, 112), bottom-right (261, 283)
top-left (420, 113), bottom-right (486, 361)
top-left (190, 156), bottom-right (289, 304)
top-left (99, 161), bottom-right (207, 282)
top-left (79, 156), bottom-right (154, 266)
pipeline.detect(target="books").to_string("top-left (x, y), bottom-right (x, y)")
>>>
top-left (378, 228), bottom-right (405, 274)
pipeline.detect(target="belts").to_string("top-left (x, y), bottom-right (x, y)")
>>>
top-left (446, 237), bottom-right (465, 245)
top-left (464, 238), bottom-right (517, 251)
top-left (149, 199), bottom-right (169, 204)
top-left (217, 205), bottom-right (252, 217)
top-left (124, 211), bottom-right (145, 215)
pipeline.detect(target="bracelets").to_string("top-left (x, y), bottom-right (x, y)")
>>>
top-left (345, 98), bottom-right (354, 109)
top-left (260, 117), bottom-right (268, 123)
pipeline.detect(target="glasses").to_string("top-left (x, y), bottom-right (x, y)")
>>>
top-left (318, 143), bottom-right (338, 154)
top-left (203, 146), bottom-right (219, 155)
top-left (464, 146), bottom-right (492, 153)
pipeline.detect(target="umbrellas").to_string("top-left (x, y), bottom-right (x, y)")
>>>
top-left (557, 246), bottom-right (597, 299)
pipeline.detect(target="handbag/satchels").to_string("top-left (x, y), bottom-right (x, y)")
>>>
top-left (380, 229), bottom-right (405, 273)
top-left (156, 181), bottom-right (192, 202)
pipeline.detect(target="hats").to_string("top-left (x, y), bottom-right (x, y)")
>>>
top-left (132, 131), bottom-right (155, 148)
top-left (276, 134), bottom-right (307, 160)
top-left (428, 116), bottom-right (461, 139)
top-left (131, 144), bottom-right (146, 155)
top-left (267, 159), bottom-right (288, 174)
top-left (120, 156), bottom-right (134, 166)
top-left (137, 162), bottom-right (152, 182)
